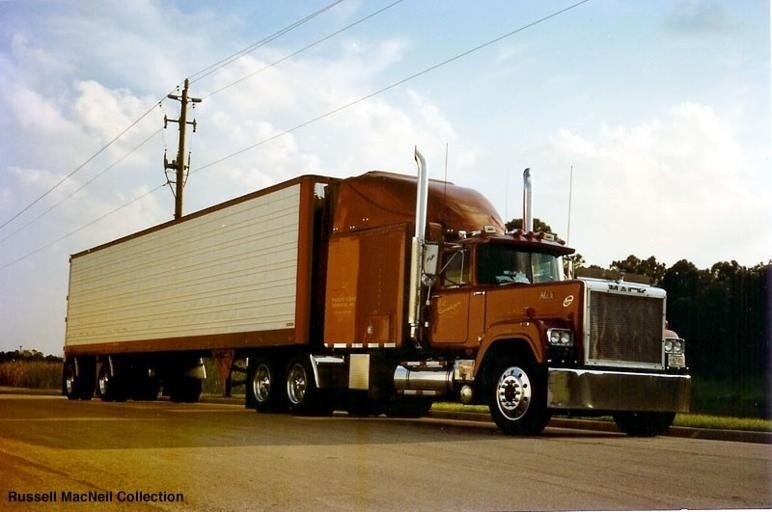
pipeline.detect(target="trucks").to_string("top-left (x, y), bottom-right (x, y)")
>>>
top-left (60, 145), bottom-right (692, 440)
